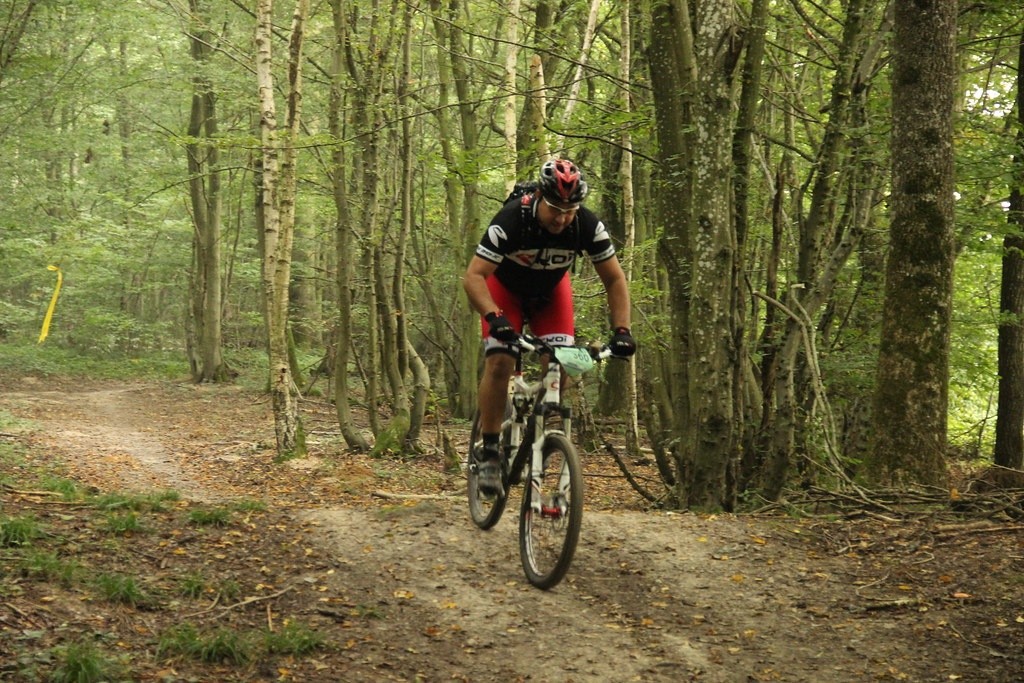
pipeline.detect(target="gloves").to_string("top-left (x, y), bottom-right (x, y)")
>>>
top-left (609, 327), bottom-right (636, 356)
top-left (485, 310), bottom-right (520, 341)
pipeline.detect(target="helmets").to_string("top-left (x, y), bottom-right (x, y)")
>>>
top-left (537, 159), bottom-right (588, 203)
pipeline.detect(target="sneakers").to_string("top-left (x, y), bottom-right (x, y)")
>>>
top-left (478, 455), bottom-right (503, 494)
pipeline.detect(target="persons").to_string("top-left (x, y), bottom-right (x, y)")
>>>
top-left (463, 159), bottom-right (638, 491)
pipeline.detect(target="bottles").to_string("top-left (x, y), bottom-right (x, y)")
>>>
top-left (528, 377), bottom-right (540, 410)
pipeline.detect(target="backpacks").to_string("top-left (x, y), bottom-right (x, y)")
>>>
top-left (502, 183), bottom-right (580, 263)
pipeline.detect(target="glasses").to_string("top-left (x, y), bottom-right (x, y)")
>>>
top-left (543, 197), bottom-right (580, 215)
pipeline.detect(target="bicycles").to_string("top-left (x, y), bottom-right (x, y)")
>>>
top-left (466, 323), bottom-right (633, 592)
